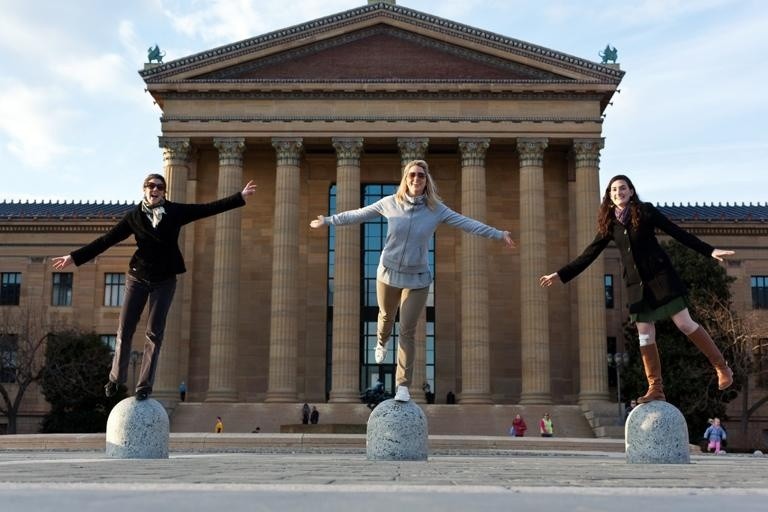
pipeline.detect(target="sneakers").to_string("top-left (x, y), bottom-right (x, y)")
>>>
top-left (375, 341), bottom-right (388, 364)
top-left (394, 384), bottom-right (410, 402)
top-left (135, 383), bottom-right (153, 401)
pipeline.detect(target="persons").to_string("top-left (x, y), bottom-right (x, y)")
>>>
top-left (250, 426), bottom-right (260, 433)
top-left (707, 416), bottom-right (730, 453)
top-left (703, 416), bottom-right (727, 456)
top-left (214, 415), bottom-right (224, 434)
top-left (301, 401), bottom-right (310, 424)
top-left (537, 411), bottom-right (554, 437)
top-left (178, 380), bottom-right (188, 401)
top-left (539, 174), bottom-right (736, 403)
top-left (49, 173), bottom-right (259, 401)
top-left (446, 386), bottom-right (456, 405)
top-left (626, 399), bottom-right (636, 418)
top-left (512, 413), bottom-right (527, 436)
top-left (423, 382), bottom-right (433, 404)
top-left (309, 158), bottom-right (516, 403)
top-left (374, 377), bottom-right (384, 394)
top-left (310, 405), bottom-right (319, 424)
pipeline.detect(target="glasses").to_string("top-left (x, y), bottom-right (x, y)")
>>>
top-left (407, 174), bottom-right (426, 180)
top-left (146, 183), bottom-right (164, 191)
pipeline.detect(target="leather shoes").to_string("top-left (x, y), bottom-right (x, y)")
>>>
top-left (104, 382), bottom-right (120, 398)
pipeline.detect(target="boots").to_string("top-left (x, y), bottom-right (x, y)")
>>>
top-left (688, 325), bottom-right (734, 391)
top-left (637, 343), bottom-right (667, 404)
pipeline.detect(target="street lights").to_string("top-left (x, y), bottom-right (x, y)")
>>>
top-left (127, 350), bottom-right (143, 396)
top-left (607, 352), bottom-right (630, 426)
